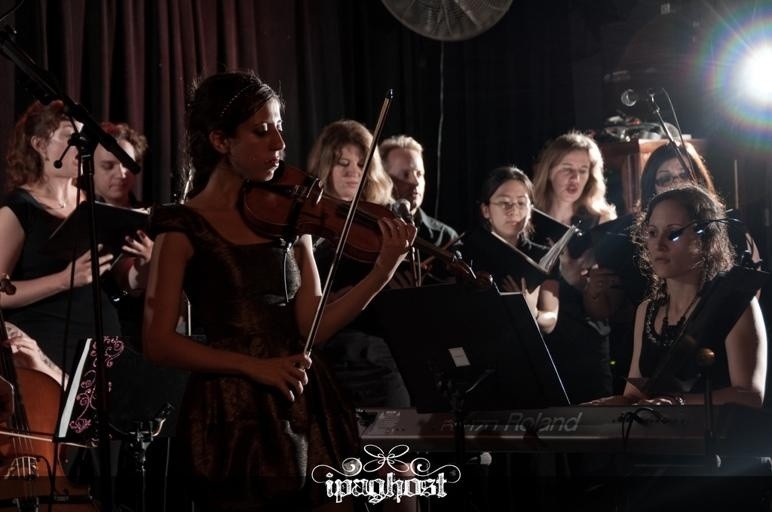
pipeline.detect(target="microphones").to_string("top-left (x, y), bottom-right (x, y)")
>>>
top-left (668, 217), bottom-right (757, 268)
top-left (621, 87), bottom-right (664, 107)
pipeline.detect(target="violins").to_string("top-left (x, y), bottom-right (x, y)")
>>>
top-left (239, 165), bottom-right (493, 291)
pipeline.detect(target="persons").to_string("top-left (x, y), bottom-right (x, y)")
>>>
top-left (0, 66), bottom-right (772, 512)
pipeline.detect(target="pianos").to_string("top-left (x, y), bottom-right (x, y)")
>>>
top-left (353, 404), bottom-right (772, 478)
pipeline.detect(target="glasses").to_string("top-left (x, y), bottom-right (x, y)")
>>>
top-left (655, 171), bottom-right (688, 187)
top-left (488, 199), bottom-right (533, 210)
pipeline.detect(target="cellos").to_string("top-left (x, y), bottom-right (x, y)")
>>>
top-left (0, 271), bottom-right (92, 502)
